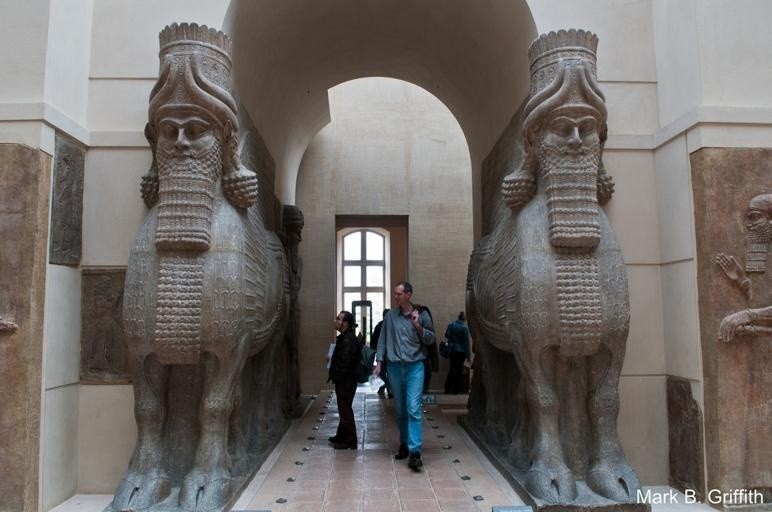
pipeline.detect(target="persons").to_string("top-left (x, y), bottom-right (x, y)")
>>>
top-left (445, 311), bottom-right (471, 394)
top-left (140, 22), bottom-right (258, 251)
top-left (716, 194), bottom-right (772, 495)
top-left (501, 29), bottom-right (615, 248)
top-left (328, 282), bottom-right (439, 468)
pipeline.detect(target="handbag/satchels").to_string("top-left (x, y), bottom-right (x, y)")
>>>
top-left (439, 343), bottom-right (452, 359)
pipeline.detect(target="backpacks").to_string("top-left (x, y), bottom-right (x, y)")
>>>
top-left (347, 336), bottom-right (376, 384)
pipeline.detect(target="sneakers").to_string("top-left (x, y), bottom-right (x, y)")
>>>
top-left (408, 452), bottom-right (423, 467)
top-left (394, 443), bottom-right (409, 459)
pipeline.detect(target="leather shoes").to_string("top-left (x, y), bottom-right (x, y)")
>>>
top-left (328, 435), bottom-right (357, 450)
